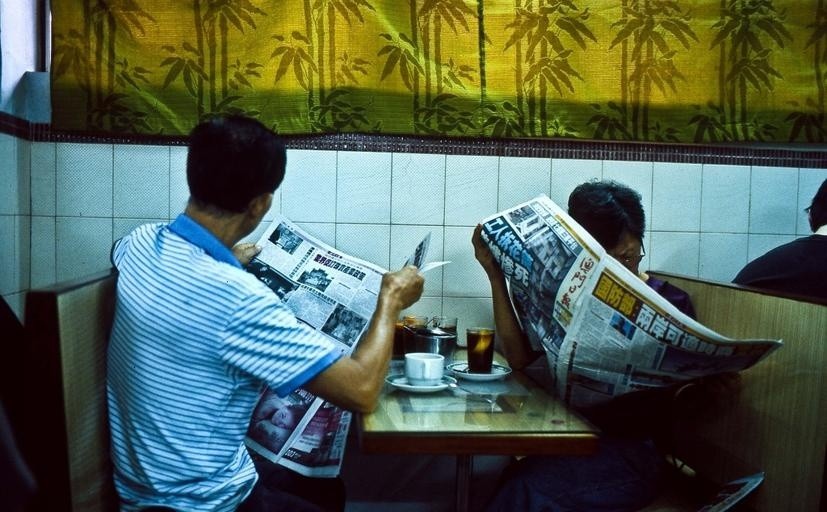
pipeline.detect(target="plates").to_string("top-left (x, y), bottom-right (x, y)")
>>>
top-left (446, 364), bottom-right (512, 382)
top-left (384, 374), bottom-right (457, 394)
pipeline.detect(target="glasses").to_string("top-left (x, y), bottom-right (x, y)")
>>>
top-left (618, 240), bottom-right (645, 268)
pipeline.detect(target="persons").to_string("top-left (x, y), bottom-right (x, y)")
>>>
top-left (245, 262), bottom-right (270, 278)
top-left (103, 113), bottom-right (424, 511)
top-left (471, 179), bottom-right (745, 510)
top-left (612, 316), bottom-right (627, 337)
top-left (731, 178), bottom-right (827, 301)
top-left (250, 394), bottom-right (310, 430)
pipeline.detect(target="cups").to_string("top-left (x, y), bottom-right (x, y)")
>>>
top-left (433, 316), bottom-right (457, 334)
top-left (404, 353), bottom-right (445, 385)
top-left (404, 317), bottom-right (428, 354)
top-left (466, 328), bottom-right (496, 373)
top-left (392, 320), bottom-right (405, 360)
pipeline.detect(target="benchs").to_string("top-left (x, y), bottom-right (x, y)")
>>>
top-left (642, 271), bottom-right (827, 512)
top-left (25, 265), bottom-right (119, 512)
